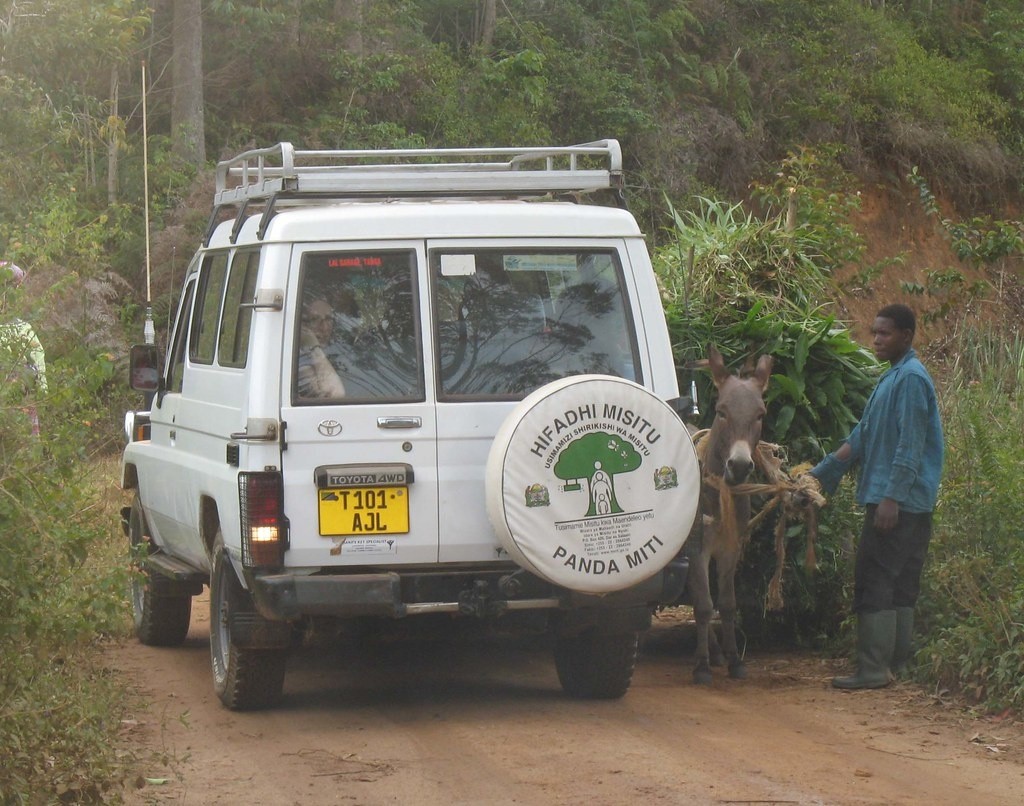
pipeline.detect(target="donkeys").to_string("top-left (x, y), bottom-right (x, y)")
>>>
top-left (661, 339), bottom-right (776, 685)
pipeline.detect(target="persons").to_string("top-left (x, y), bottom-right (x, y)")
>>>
top-left (798, 304), bottom-right (943, 690)
top-left (298, 292), bottom-right (346, 409)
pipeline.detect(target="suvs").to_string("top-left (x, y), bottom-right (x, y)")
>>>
top-left (118, 136), bottom-right (711, 720)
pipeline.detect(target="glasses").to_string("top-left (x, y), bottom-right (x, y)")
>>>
top-left (301, 316), bottom-right (336, 327)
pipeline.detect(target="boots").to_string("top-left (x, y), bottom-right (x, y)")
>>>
top-left (889, 606), bottom-right (915, 680)
top-left (832, 611), bottom-right (894, 688)
